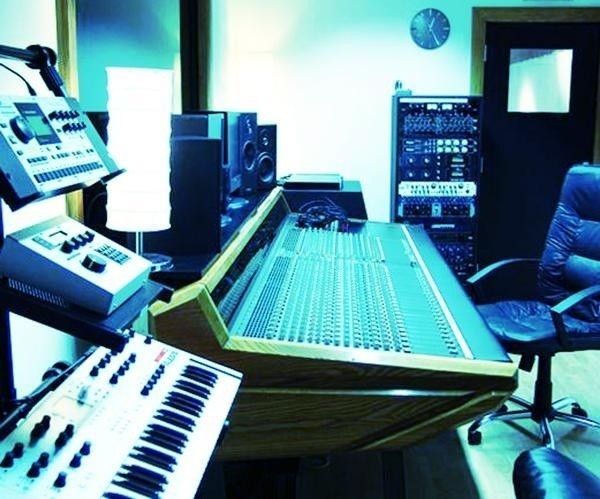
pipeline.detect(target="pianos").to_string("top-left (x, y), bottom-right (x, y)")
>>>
top-left (0, 328), bottom-right (244, 499)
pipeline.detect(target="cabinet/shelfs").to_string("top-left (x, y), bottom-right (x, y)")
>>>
top-left (390, 81), bottom-right (484, 296)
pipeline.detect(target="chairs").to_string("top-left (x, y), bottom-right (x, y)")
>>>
top-left (465, 164), bottom-right (600, 451)
top-left (512, 448), bottom-right (600, 499)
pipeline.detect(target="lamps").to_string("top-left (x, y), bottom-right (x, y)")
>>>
top-left (106, 67), bottom-right (174, 272)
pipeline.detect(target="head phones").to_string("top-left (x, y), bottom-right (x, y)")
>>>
top-left (298, 198), bottom-right (349, 230)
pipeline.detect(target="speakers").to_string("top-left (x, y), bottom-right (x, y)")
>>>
top-left (229, 111), bottom-right (256, 192)
top-left (257, 124), bottom-right (279, 187)
top-left (171, 137), bottom-right (222, 255)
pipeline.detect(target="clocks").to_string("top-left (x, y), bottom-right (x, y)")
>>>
top-left (410, 9), bottom-right (450, 49)
